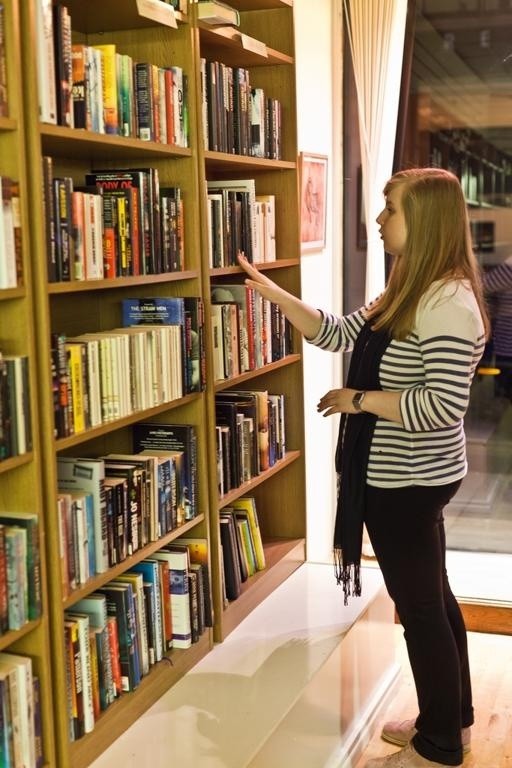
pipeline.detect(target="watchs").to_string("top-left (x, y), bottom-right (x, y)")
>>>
top-left (353, 388), bottom-right (372, 414)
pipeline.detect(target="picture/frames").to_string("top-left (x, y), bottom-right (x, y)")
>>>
top-left (298, 152), bottom-right (328, 253)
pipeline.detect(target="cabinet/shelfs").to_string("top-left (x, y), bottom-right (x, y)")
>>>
top-left (25, 0), bottom-right (218, 768)
top-left (196, 2), bottom-right (308, 651)
top-left (0, 1), bottom-right (58, 768)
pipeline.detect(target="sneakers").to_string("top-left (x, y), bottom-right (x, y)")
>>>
top-left (378, 715), bottom-right (474, 756)
top-left (362, 741), bottom-right (463, 768)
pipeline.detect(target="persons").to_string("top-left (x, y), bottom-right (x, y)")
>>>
top-left (481, 250), bottom-right (512, 403)
top-left (235, 166), bottom-right (490, 767)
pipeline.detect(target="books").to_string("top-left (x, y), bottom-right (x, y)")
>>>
top-left (214, 390), bottom-right (285, 496)
top-left (56, 423), bottom-right (199, 602)
top-left (0, 648), bottom-right (46, 768)
top-left (0, 357), bottom-right (33, 462)
top-left (1, 177), bottom-right (25, 288)
top-left (198, 0), bottom-right (241, 28)
top-left (51, 297), bottom-right (207, 441)
top-left (36, 0), bottom-right (189, 148)
top-left (0, 511), bottom-right (43, 636)
top-left (220, 497), bottom-right (265, 602)
top-left (42, 157), bottom-right (184, 281)
top-left (212, 284), bottom-right (293, 380)
top-left (205, 180), bottom-right (276, 268)
top-left (62, 538), bottom-right (213, 742)
top-left (199, 58), bottom-right (281, 162)
